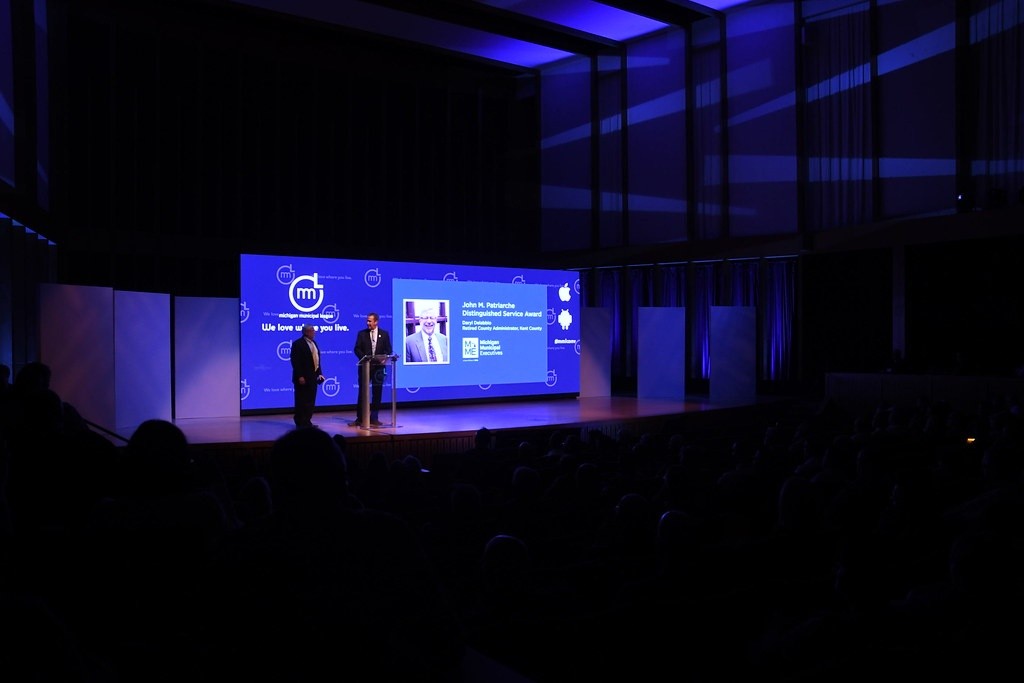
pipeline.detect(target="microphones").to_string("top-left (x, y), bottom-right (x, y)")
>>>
top-left (373, 340), bottom-right (375, 342)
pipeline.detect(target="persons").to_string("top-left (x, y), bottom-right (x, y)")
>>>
top-left (0, 363), bottom-right (1024, 683)
top-left (348, 312), bottom-right (392, 426)
top-left (290, 326), bottom-right (324, 428)
top-left (406, 310), bottom-right (448, 362)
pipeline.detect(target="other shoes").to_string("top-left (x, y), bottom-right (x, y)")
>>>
top-left (348, 418), bottom-right (361, 426)
top-left (296, 423), bottom-right (318, 429)
top-left (370, 420), bottom-right (383, 425)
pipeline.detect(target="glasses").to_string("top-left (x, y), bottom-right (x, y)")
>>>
top-left (420, 316), bottom-right (436, 320)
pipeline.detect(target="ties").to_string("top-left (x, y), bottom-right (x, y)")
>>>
top-left (428, 337), bottom-right (437, 362)
top-left (372, 331), bottom-right (376, 357)
top-left (310, 341), bottom-right (318, 371)
